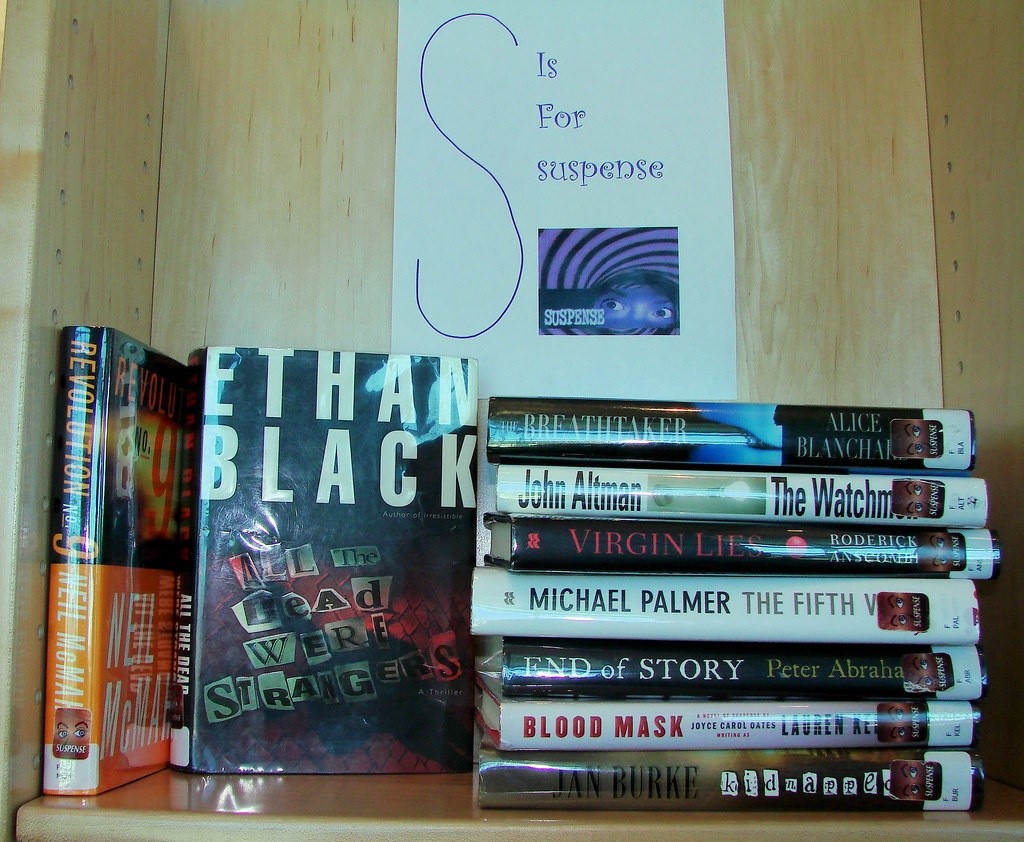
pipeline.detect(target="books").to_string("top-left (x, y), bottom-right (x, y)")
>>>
top-left (40, 325), bottom-right (1005, 813)
